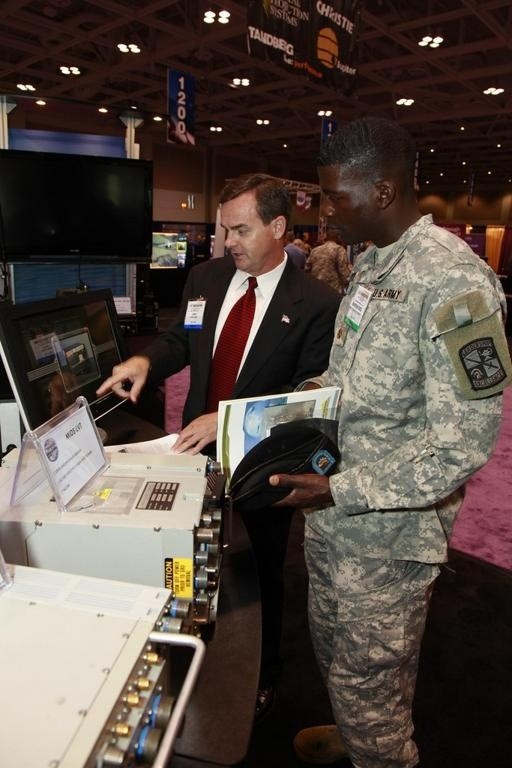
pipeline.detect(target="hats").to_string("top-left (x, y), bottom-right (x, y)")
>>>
top-left (228, 427), bottom-right (342, 517)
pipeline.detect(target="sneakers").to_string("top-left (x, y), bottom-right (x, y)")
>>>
top-left (293, 725), bottom-right (349, 766)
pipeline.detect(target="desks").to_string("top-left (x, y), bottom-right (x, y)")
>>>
top-left (96, 402), bottom-right (262, 766)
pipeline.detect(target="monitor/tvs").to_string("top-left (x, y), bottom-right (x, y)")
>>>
top-left (0, 289), bottom-right (139, 443)
top-left (149, 231), bottom-right (189, 271)
top-left (0, 149), bottom-right (153, 262)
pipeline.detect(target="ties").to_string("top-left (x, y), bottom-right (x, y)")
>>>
top-left (205, 276), bottom-right (257, 408)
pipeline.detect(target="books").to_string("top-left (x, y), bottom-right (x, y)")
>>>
top-left (215, 384), bottom-right (342, 497)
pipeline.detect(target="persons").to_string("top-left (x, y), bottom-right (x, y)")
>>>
top-left (268, 113), bottom-right (511, 768)
top-left (286, 227), bottom-right (314, 254)
top-left (304, 225), bottom-right (351, 294)
top-left (96, 172), bottom-right (343, 724)
